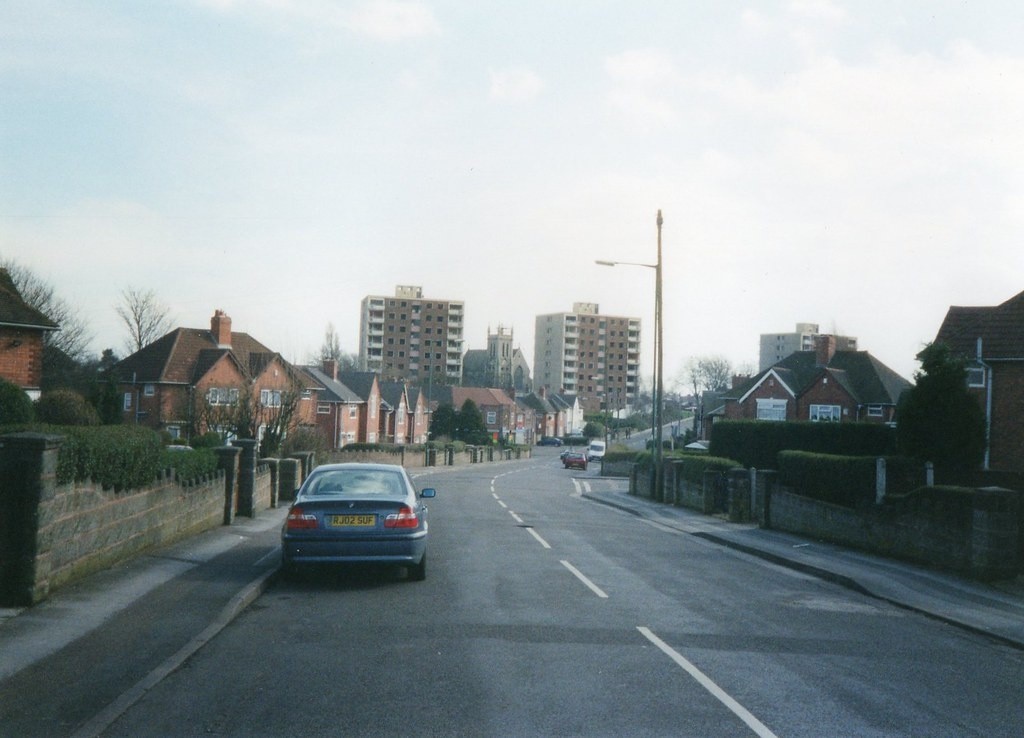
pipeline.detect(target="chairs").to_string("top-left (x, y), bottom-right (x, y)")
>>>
top-left (320, 482), bottom-right (343, 491)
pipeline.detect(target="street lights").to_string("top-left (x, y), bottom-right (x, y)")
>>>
top-left (596, 258), bottom-right (666, 505)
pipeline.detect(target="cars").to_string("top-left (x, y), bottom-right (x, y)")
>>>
top-left (536, 437), bottom-right (564, 447)
top-left (282, 460), bottom-right (436, 582)
top-left (561, 450), bottom-right (587, 470)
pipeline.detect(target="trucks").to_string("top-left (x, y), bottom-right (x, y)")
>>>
top-left (587, 440), bottom-right (605, 462)
